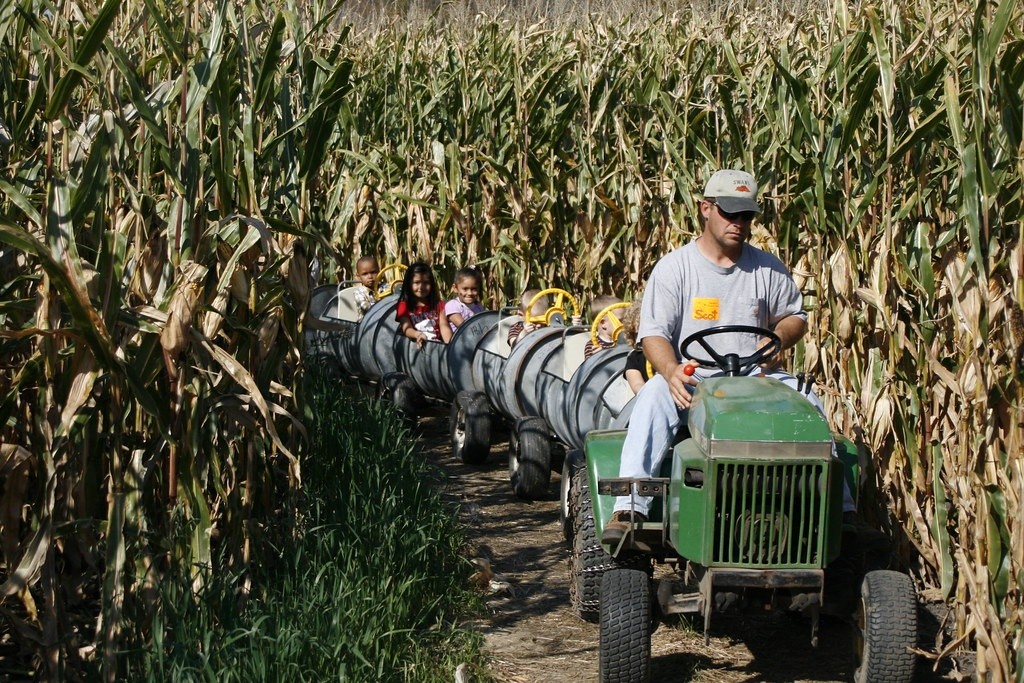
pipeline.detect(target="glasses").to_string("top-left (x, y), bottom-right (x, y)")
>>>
top-left (708, 200), bottom-right (754, 220)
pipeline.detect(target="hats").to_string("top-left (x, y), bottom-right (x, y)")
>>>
top-left (703, 169), bottom-right (761, 213)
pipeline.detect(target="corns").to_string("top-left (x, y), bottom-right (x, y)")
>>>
top-left (132, 229), bottom-right (310, 360)
top-left (790, 243), bottom-right (855, 297)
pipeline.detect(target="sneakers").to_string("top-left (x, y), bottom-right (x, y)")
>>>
top-left (602, 509), bottom-right (644, 543)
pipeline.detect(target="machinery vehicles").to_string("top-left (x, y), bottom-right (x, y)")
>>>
top-left (571, 324), bottom-right (917, 683)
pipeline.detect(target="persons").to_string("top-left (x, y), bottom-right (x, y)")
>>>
top-left (353, 255), bottom-right (391, 323)
top-left (444, 268), bottom-right (484, 332)
top-left (584, 295), bottom-right (625, 360)
top-left (601, 171), bottom-right (889, 548)
top-left (507, 289), bottom-right (581, 350)
top-left (395, 262), bottom-right (453, 350)
